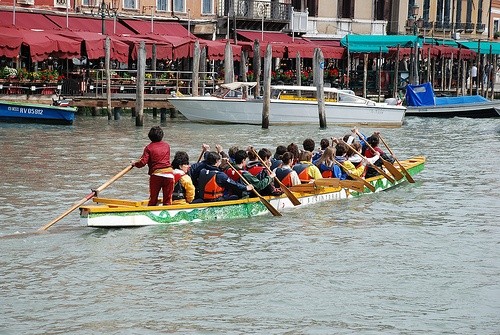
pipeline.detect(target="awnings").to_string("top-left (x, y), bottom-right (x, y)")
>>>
top-left (0, 9), bottom-right (500, 65)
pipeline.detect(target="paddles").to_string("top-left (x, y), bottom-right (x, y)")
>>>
top-left (197, 146), bottom-right (206, 163)
top-left (251, 147), bottom-right (301, 206)
top-left (40, 164), bottom-right (134, 231)
top-left (301, 178), bottom-right (339, 188)
top-left (226, 161), bottom-right (282, 216)
top-left (276, 183), bottom-right (314, 191)
top-left (318, 137), bottom-right (394, 193)
top-left (351, 128), bottom-right (404, 181)
top-left (373, 132), bottom-right (416, 183)
top-left (340, 180), bottom-right (364, 192)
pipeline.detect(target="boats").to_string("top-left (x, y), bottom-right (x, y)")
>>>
top-left (168, 81), bottom-right (408, 129)
top-left (80, 157), bottom-right (426, 228)
top-left (379, 84), bottom-right (500, 119)
top-left (0, 103), bottom-right (79, 127)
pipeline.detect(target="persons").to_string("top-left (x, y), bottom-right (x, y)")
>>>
top-left (171, 134), bottom-right (396, 205)
top-left (357, 61), bottom-right (496, 88)
top-left (132, 126), bottom-right (175, 206)
top-left (21, 60), bottom-right (224, 92)
